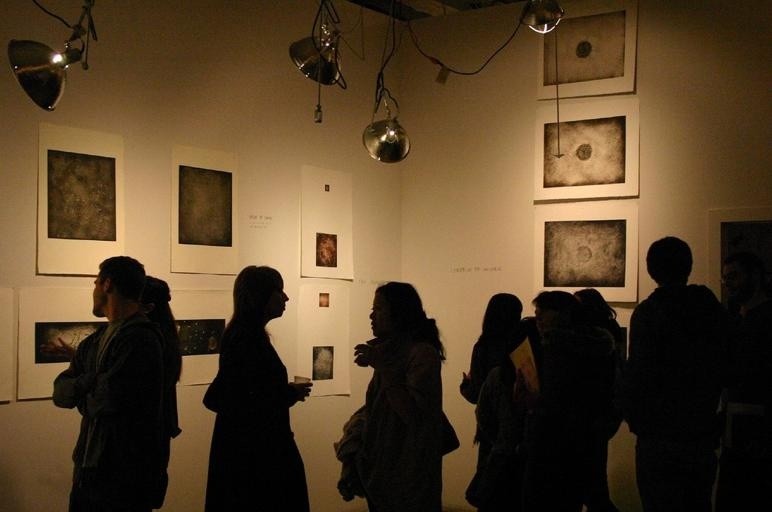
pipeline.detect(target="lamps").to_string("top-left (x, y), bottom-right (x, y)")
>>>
top-left (362, 0), bottom-right (411, 164)
top-left (4, 0), bottom-right (97, 113)
top-left (519, 0), bottom-right (565, 161)
top-left (288, 0), bottom-right (348, 125)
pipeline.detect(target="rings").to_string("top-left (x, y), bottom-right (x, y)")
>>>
top-left (304, 387), bottom-right (307, 394)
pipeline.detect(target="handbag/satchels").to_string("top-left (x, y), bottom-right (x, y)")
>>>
top-left (442, 412), bottom-right (460, 456)
top-left (203, 370), bottom-right (221, 414)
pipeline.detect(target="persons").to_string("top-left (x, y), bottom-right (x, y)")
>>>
top-left (516, 291), bottom-right (617, 512)
top-left (715, 251), bottom-right (772, 512)
top-left (574, 289), bottom-right (620, 512)
top-left (466, 317), bottom-right (544, 512)
top-left (204, 267), bottom-right (313, 512)
top-left (460, 293), bottom-right (522, 472)
top-left (59, 276), bottom-right (181, 512)
top-left (334, 283), bottom-right (448, 512)
top-left (620, 237), bottom-right (728, 512)
top-left (52, 256), bottom-right (162, 512)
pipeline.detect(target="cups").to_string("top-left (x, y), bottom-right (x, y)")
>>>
top-left (294, 376), bottom-right (313, 385)
